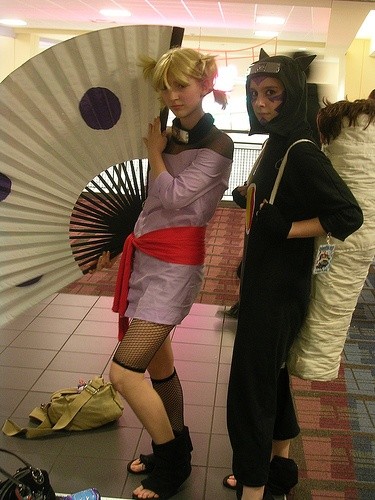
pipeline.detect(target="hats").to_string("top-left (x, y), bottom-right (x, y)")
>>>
top-left (245, 47), bottom-right (317, 136)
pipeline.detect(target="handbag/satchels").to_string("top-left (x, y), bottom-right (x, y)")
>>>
top-left (0, 449), bottom-right (57, 500)
top-left (2, 377), bottom-right (124, 439)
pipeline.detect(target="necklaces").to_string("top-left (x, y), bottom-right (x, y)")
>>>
top-left (171, 113), bottom-right (215, 145)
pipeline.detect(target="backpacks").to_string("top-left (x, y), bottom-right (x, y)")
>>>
top-left (244, 87), bottom-right (375, 382)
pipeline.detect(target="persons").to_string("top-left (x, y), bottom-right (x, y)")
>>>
top-left (88, 47), bottom-right (234, 500)
top-left (222, 48), bottom-right (364, 500)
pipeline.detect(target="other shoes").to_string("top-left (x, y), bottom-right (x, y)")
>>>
top-left (132, 435), bottom-right (192, 500)
top-left (223, 455), bottom-right (299, 495)
top-left (127, 425), bottom-right (193, 474)
top-left (215, 302), bottom-right (239, 319)
